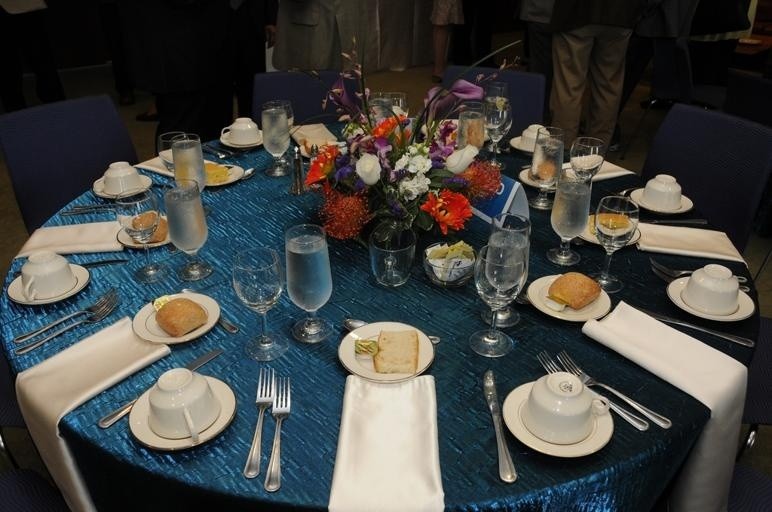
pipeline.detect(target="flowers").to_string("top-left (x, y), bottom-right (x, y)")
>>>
top-left (282, 37), bottom-right (524, 223)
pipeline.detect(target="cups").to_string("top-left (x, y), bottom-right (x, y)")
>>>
top-left (366, 92), bottom-right (410, 120)
top-left (103, 161), bottom-right (141, 196)
top-left (520, 372), bottom-right (610, 445)
top-left (679, 263), bottom-right (739, 315)
top-left (367, 227), bottom-right (416, 290)
top-left (148, 366), bottom-right (218, 449)
top-left (521, 124), bottom-right (548, 151)
top-left (222, 117), bottom-right (261, 145)
top-left (643, 172), bottom-right (683, 209)
top-left (19, 251), bottom-right (76, 304)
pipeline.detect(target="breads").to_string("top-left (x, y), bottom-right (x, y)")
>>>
top-left (528, 162), bottom-right (555, 182)
top-left (548, 271), bottom-right (601, 311)
top-left (155, 297), bottom-right (208, 338)
top-left (132, 212), bottom-right (168, 244)
top-left (373, 330), bottom-right (419, 374)
top-left (589, 213), bottom-right (630, 235)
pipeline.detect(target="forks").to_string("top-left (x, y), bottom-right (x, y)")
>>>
top-left (263, 375), bottom-right (292, 491)
top-left (15, 288), bottom-right (124, 358)
top-left (59, 202), bottom-right (129, 216)
top-left (537, 349), bottom-right (650, 436)
top-left (200, 141), bottom-right (252, 161)
top-left (554, 349), bottom-right (673, 430)
top-left (242, 366), bottom-right (276, 479)
top-left (643, 254), bottom-right (750, 294)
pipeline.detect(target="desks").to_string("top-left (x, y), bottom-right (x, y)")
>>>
top-left (2, 121), bottom-right (759, 511)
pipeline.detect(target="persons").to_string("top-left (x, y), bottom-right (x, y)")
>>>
top-left (3, 3), bottom-right (757, 166)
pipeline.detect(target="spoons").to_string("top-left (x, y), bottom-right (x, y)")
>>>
top-left (240, 167), bottom-right (257, 181)
top-left (344, 317), bottom-right (440, 345)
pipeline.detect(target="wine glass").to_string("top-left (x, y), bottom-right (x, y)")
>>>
top-left (467, 210), bottom-right (534, 358)
top-left (113, 131), bottom-right (215, 285)
top-left (231, 249), bottom-right (289, 363)
top-left (283, 225), bottom-right (338, 347)
top-left (258, 99), bottom-right (295, 179)
top-left (458, 80), bottom-right (512, 172)
top-left (528, 127), bottom-right (640, 297)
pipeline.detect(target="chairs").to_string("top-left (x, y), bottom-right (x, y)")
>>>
top-left (637, 103), bottom-right (769, 274)
top-left (730, 315), bottom-right (771, 469)
top-left (443, 66), bottom-right (546, 139)
top-left (718, 460), bottom-right (771, 509)
top-left (251, 70), bottom-right (364, 130)
top-left (2, 95), bottom-right (143, 235)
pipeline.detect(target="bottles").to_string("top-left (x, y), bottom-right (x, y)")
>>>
top-left (291, 145), bottom-right (324, 198)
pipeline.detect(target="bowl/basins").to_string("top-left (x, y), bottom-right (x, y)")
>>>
top-left (420, 243), bottom-right (479, 288)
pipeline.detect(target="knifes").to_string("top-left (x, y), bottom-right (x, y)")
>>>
top-left (98, 345), bottom-right (226, 433)
top-left (476, 367), bottom-right (517, 485)
top-left (644, 218), bottom-right (708, 226)
top-left (85, 258), bottom-right (127, 270)
top-left (639, 308), bottom-right (757, 350)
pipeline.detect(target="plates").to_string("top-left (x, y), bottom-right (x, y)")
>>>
top-left (525, 272), bottom-right (610, 327)
top-left (420, 118), bottom-right (458, 138)
top-left (630, 186), bottom-right (697, 214)
top-left (129, 374), bottom-right (238, 452)
top-left (133, 292), bottom-right (220, 345)
top-left (337, 318), bottom-right (435, 387)
top-left (5, 260), bottom-right (92, 308)
top-left (509, 137), bottom-right (526, 152)
top-left (92, 166), bottom-right (238, 202)
top-left (221, 132), bottom-right (264, 149)
top-left (662, 274), bottom-right (757, 323)
top-left (502, 378), bottom-right (616, 459)
top-left (518, 168), bottom-right (557, 190)
